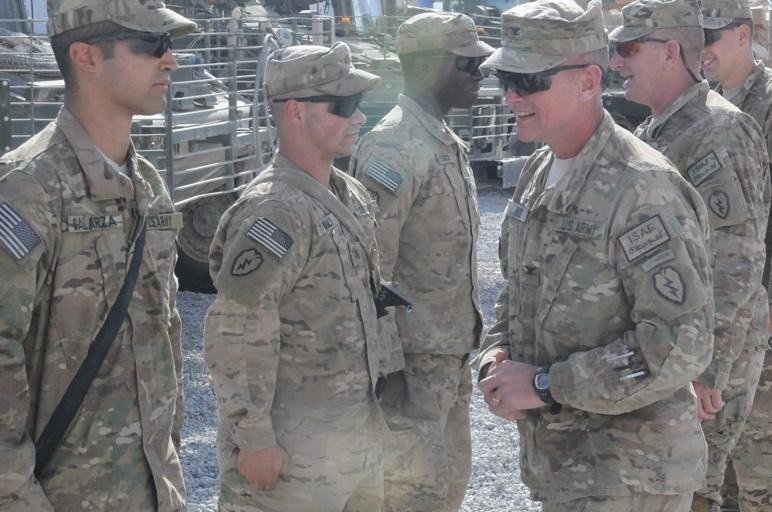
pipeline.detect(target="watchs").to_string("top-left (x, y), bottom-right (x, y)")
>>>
top-left (533, 363), bottom-right (562, 415)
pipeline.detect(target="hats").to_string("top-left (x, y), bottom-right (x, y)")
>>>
top-left (46, 1), bottom-right (197, 41)
top-left (607, 1), bottom-right (702, 43)
top-left (396, 11), bottom-right (495, 58)
top-left (702, 0), bottom-right (753, 31)
top-left (263, 39), bottom-right (382, 100)
top-left (478, 0), bottom-right (610, 79)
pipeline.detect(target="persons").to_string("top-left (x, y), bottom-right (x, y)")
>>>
top-left (347, 9), bottom-right (495, 512)
top-left (605, 0), bottom-right (771, 512)
top-left (700, 0), bottom-right (772, 512)
top-left (201, 39), bottom-right (411, 512)
top-left (0, 0), bottom-right (200, 512)
top-left (468, 0), bottom-right (716, 512)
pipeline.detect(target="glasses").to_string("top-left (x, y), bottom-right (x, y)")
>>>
top-left (613, 38), bottom-right (667, 58)
top-left (454, 54), bottom-right (481, 75)
top-left (271, 93), bottom-right (365, 119)
top-left (703, 22), bottom-right (749, 48)
top-left (61, 32), bottom-right (174, 59)
top-left (495, 64), bottom-right (600, 98)
